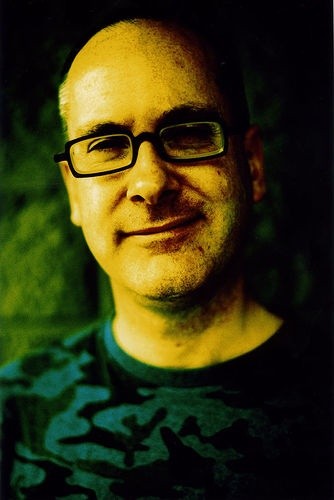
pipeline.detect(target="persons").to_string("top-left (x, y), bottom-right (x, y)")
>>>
top-left (0, 18), bottom-right (334, 499)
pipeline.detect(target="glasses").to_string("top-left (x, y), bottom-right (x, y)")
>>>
top-left (55, 115), bottom-right (250, 175)
